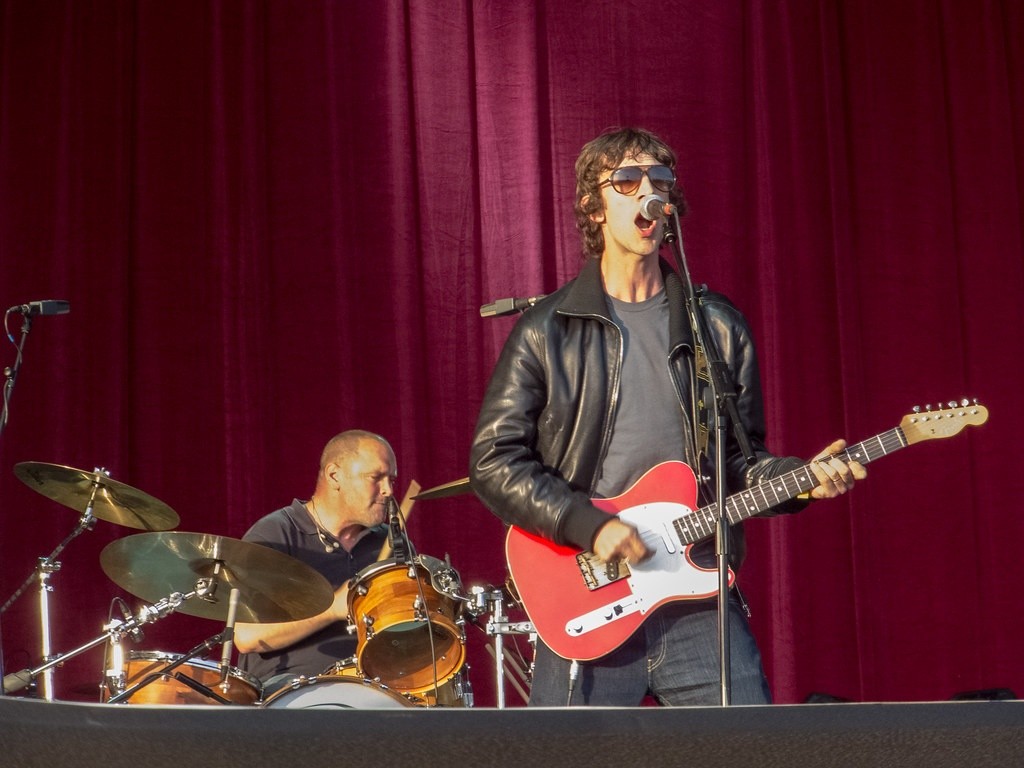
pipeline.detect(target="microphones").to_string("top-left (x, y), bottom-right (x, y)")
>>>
top-left (29, 300), bottom-right (71, 316)
top-left (480, 297), bottom-right (530, 318)
top-left (639, 194), bottom-right (677, 221)
top-left (387, 500), bottom-right (403, 549)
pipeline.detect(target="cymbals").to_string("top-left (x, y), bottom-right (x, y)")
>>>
top-left (408, 476), bottom-right (474, 499)
top-left (101, 532), bottom-right (335, 624)
top-left (13, 461), bottom-right (180, 532)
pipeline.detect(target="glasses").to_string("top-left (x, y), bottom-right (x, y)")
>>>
top-left (591, 165), bottom-right (677, 194)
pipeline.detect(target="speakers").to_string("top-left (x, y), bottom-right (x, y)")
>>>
top-left (1, 697), bottom-right (1024, 768)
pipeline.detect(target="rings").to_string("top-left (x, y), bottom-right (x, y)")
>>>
top-left (833, 476), bottom-right (842, 484)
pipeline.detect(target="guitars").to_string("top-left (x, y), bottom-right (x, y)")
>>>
top-left (505, 398), bottom-right (989, 662)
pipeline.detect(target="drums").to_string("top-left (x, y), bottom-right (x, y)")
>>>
top-left (255, 674), bottom-right (418, 707)
top-left (100, 651), bottom-right (261, 706)
top-left (346, 556), bottom-right (465, 695)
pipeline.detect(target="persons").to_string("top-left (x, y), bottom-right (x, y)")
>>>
top-left (230, 430), bottom-right (417, 701)
top-left (468, 133), bottom-right (868, 707)
